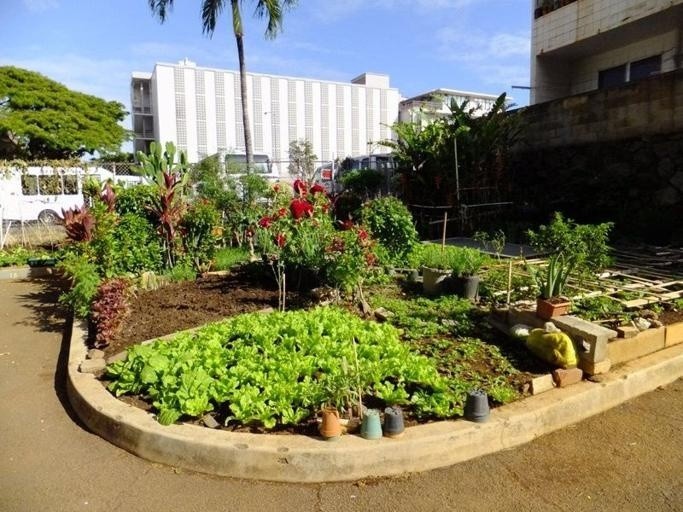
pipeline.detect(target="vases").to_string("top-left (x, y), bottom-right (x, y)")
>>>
top-left (320, 391), bottom-right (490, 440)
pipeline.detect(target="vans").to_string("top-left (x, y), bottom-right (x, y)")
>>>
top-left (308, 153), bottom-right (402, 197)
top-left (184, 150), bottom-right (281, 207)
top-left (1, 165), bottom-right (118, 226)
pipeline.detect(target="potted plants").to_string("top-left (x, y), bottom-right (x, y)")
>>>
top-left (522, 245), bottom-right (575, 320)
top-left (450, 247), bottom-right (484, 299)
top-left (421, 244), bottom-right (452, 293)
